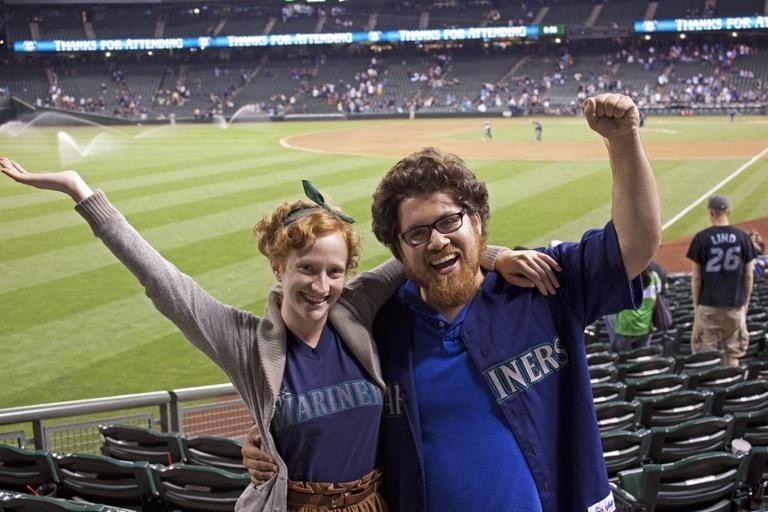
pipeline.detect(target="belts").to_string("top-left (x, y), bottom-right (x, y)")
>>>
top-left (289, 488), bottom-right (374, 509)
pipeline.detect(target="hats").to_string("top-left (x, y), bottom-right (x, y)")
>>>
top-left (708, 195), bottom-right (729, 210)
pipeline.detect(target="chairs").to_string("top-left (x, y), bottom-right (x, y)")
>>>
top-left (0, 279), bottom-right (768, 511)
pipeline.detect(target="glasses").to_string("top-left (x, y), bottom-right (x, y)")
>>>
top-left (397, 206), bottom-right (466, 246)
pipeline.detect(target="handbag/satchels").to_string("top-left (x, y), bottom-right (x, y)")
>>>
top-left (654, 294), bottom-right (672, 331)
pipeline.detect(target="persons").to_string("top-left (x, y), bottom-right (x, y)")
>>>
top-left (481, 121), bottom-right (494, 141)
top-left (0, 142), bottom-right (564, 512)
top-left (685, 195), bottom-right (768, 366)
top-left (1, 1), bottom-right (768, 126)
top-left (603, 261), bottom-right (673, 352)
top-left (532, 120), bottom-right (542, 142)
top-left (241, 92), bottom-right (662, 512)
